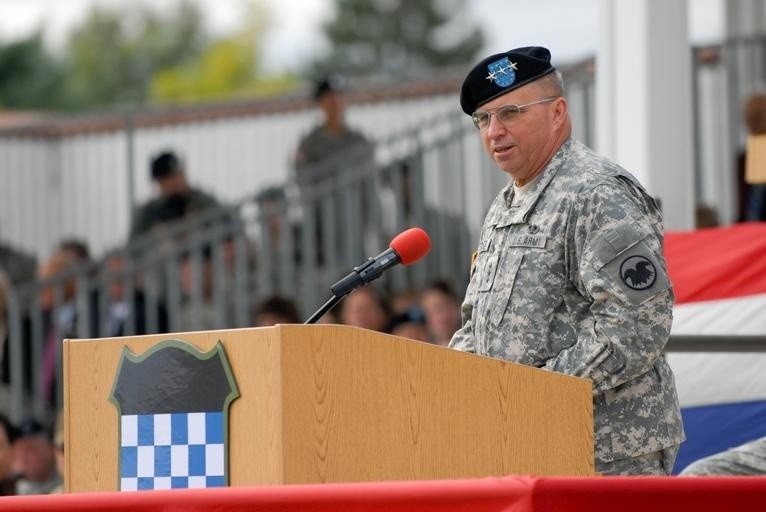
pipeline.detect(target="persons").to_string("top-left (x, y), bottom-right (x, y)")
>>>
top-left (297, 74), bottom-right (470, 345)
top-left (449, 46), bottom-right (687, 476)
top-left (0, 238), bottom-right (169, 495)
top-left (127, 150), bottom-right (306, 336)
top-left (739, 93), bottom-right (766, 223)
top-left (678, 436), bottom-right (766, 476)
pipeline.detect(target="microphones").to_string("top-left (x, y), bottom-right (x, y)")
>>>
top-left (330, 228), bottom-right (432, 297)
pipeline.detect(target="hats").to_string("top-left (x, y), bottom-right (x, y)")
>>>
top-left (460, 47), bottom-right (556, 114)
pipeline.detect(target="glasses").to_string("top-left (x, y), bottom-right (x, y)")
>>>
top-left (472, 98), bottom-right (558, 132)
